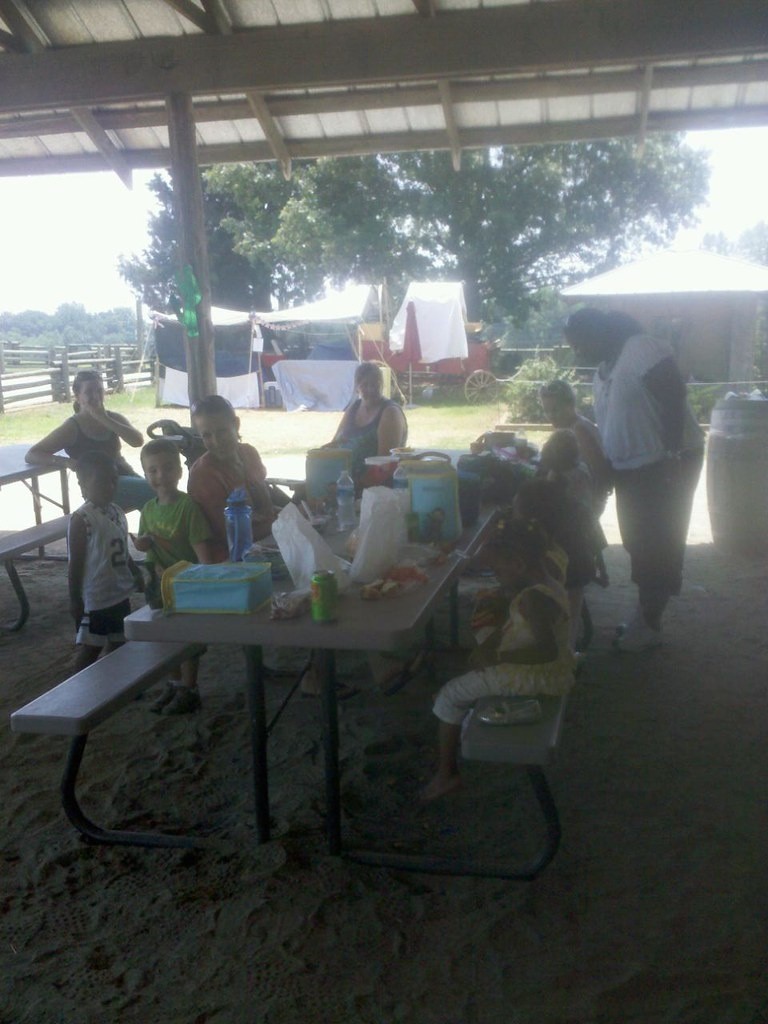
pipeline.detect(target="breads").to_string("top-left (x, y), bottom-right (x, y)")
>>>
top-left (359, 579), bottom-right (398, 602)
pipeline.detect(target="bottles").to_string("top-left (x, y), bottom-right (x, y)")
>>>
top-left (336, 472), bottom-right (356, 531)
top-left (224, 488), bottom-right (253, 559)
top-left (393, 463), bottom-right (408, 489)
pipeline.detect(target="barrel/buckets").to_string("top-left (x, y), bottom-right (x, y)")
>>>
top-left (707, 399), bottom-right (768, 553)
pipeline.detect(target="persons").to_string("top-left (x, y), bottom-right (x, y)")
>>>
top-left (187, 395), bottom-right (361, 701)
top-left (129, 440), bottom-right (215, 714)
top-left (321, 363), bottom-right (407, 462)
top-left (565, 307), bottom-right (707, 654)
top-left (67, 451), bottom-right (145, 672)
top-left (465, 380), bottom-right (614, 651)
top-left (25, 370), bottom-right (156, 514)
top-left (417, 527), bottom-right (576, 805)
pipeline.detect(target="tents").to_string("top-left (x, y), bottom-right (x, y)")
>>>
top-left (149, 285), bottom-right (380, 412)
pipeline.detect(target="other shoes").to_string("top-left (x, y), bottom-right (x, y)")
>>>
top-left (150, 681), bottom-right (201, 715)
top-left (299, 670), bottom-right (357, 700)
top-left (618, 623), bottom-right (663, 652)
top-left (478, 699), bottom-right (543, 725)
top-left (272, 645), bottom-right (308, 677)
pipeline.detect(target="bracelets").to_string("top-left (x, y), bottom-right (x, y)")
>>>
top-left (53, 456), bottom-right (60, 464)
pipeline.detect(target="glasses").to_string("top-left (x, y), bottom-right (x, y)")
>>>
top-left (190, 396), bottom-right (232, 417)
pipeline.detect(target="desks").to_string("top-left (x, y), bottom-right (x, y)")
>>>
top-left (62, 449), bottom-right (502, 879)
top-left (0, 443), bottom-right (73, 633)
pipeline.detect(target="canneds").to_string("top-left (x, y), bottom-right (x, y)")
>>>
top-left (310, 569), bottom-right (337, 623)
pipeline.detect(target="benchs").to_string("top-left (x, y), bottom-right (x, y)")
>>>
top-left (10, 637), bottom-right (195, 850)
top-left (0, 511), bottom-right (72, 632)
top-left (460, 576), bottom-right (588, 876)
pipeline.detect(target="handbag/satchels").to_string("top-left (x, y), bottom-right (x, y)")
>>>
top-left (306, 448), bottom-right (352, 507)
top-left (271, 502), bottom-right (349, 600)
top-left (393, 460), bottom-right (463, 546)
top-left (349, 486), bottom-right (409, 583)
top-left (161, 560), bottom-right (271, 616)
